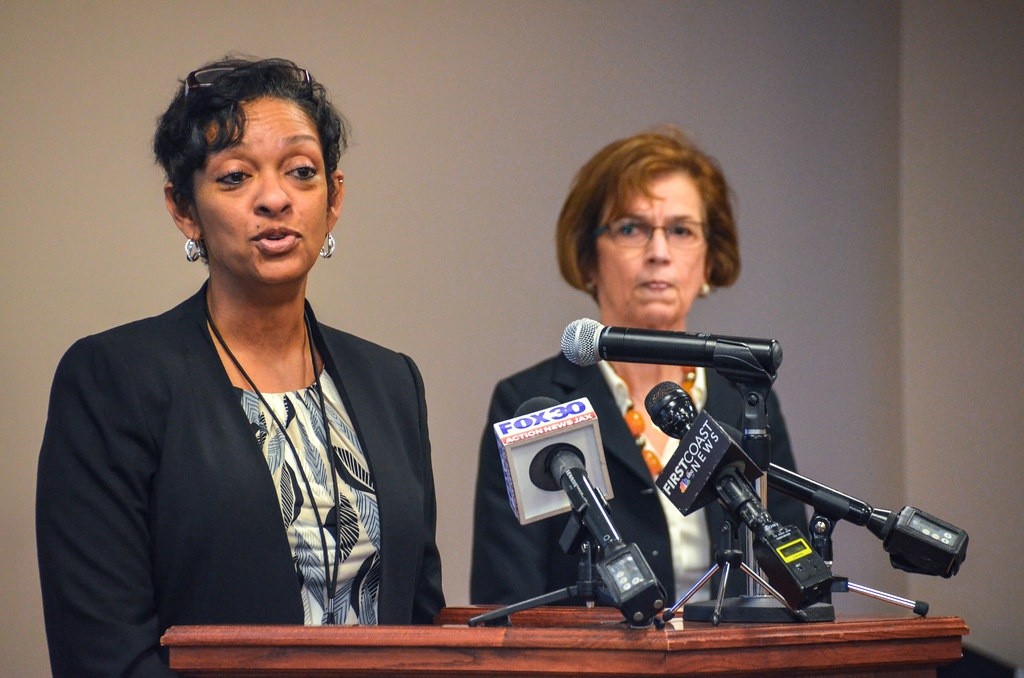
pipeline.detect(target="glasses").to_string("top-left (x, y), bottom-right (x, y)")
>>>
top-left (594, 212), bottom-right (711, 249)
top-left (182, 65), bottom-right (313, 102)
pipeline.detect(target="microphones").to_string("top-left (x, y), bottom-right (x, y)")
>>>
top-left (488, 317), bottom-right (970, 627)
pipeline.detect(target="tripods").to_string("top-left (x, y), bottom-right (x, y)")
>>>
top-left (804, 484), bottom-right (929, 616)
top-left (663, 493), bottom-right (808, 627)
top-left (468, 487), bottom-right (673, 631)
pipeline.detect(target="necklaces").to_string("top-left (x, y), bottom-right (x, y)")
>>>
top-left (622, 364), bottom-right (697, 480)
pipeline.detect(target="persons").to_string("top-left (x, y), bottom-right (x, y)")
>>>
top-left (471, 128), bottom-right (834, 605)
top-left (35, 51), bottom-right (446, 677)
